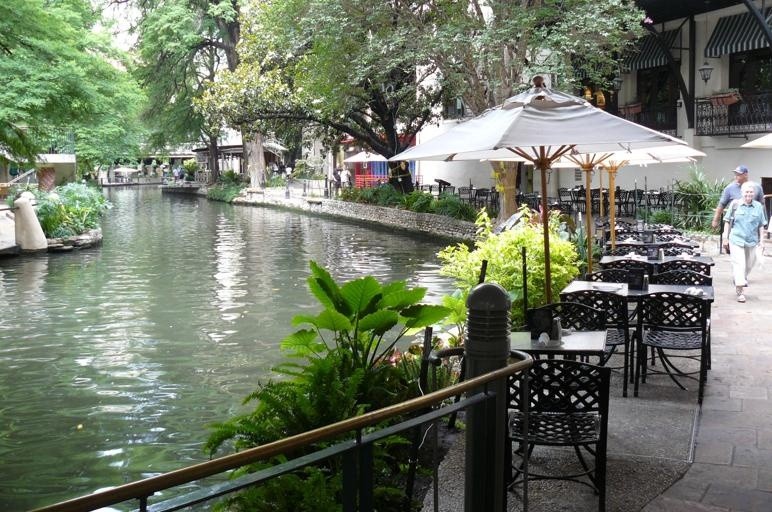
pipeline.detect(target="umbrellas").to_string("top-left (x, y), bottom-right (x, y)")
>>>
top-left (739, 132), bottom-right (772, 149)
top-left (342, 150), bottom-right (388, 187)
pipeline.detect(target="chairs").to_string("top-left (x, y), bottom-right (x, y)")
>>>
top-left (417, 185), bottom-right (681, 211)
top-left (560, 216), bottom-right (714, 407)
top-left (526, 302), bottom-right (606, 391)
top-left (507, 359), bottom-right (611, 511)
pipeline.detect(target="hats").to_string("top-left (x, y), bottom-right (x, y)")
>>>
top-left (732, 166), bottom-right (749, 174)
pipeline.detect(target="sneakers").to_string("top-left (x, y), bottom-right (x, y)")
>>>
top-left (738, 294), bottom-right (746, 302)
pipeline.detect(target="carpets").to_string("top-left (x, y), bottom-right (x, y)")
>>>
top-left (419, 336), bottom-right (703, 512)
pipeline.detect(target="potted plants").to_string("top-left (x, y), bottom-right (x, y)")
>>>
top-left (709, 87), bottom-right (741, 106)
top-left (617, 98), bottom-right (642, 114)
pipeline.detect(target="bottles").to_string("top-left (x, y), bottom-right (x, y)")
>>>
top-left (539, 332), bottom-right (550, 346)
top-left (652, 234), bottom-right (657, 244)
top-left (658, 249), bottom-right (665, 260)
top-left (643, 275), bottom-right (649, 289)
top-left (552, 316), bottom-right (562, 338)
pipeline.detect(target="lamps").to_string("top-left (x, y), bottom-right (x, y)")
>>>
top-left (698, 61), bottom-right (714, 86)
top-left (613, 77), bottom-right (624, 91)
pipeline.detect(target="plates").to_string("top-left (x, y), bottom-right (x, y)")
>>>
top-left (684, 290), bottom-right (706, 296)
top-left (624, 254), bottom-right (641, 258)
top-left (623, 239), bottom-right (636, 244)
top-left (593, 283), bottom-right (622, 292)
top-left (561, 327), bottom-right (572, 335)
top-left (677, 254), bottom-right (693, 258)
top-left (535, 341), bottom-right (564, 348)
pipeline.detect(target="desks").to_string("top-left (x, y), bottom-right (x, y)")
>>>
top-left (507, 331), bottom-right (608, 456)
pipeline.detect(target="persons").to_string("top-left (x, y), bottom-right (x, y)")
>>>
top-left (340, 165), bottom-right (358, 191)
top-left (269, 161), bottom-right (295, 178)
top-left (157, 161), bottom-right (191, 185)
top-left (710, 164), bottom-right (766, 228)
top-left (722, 181), bottom-right (767, 302)
